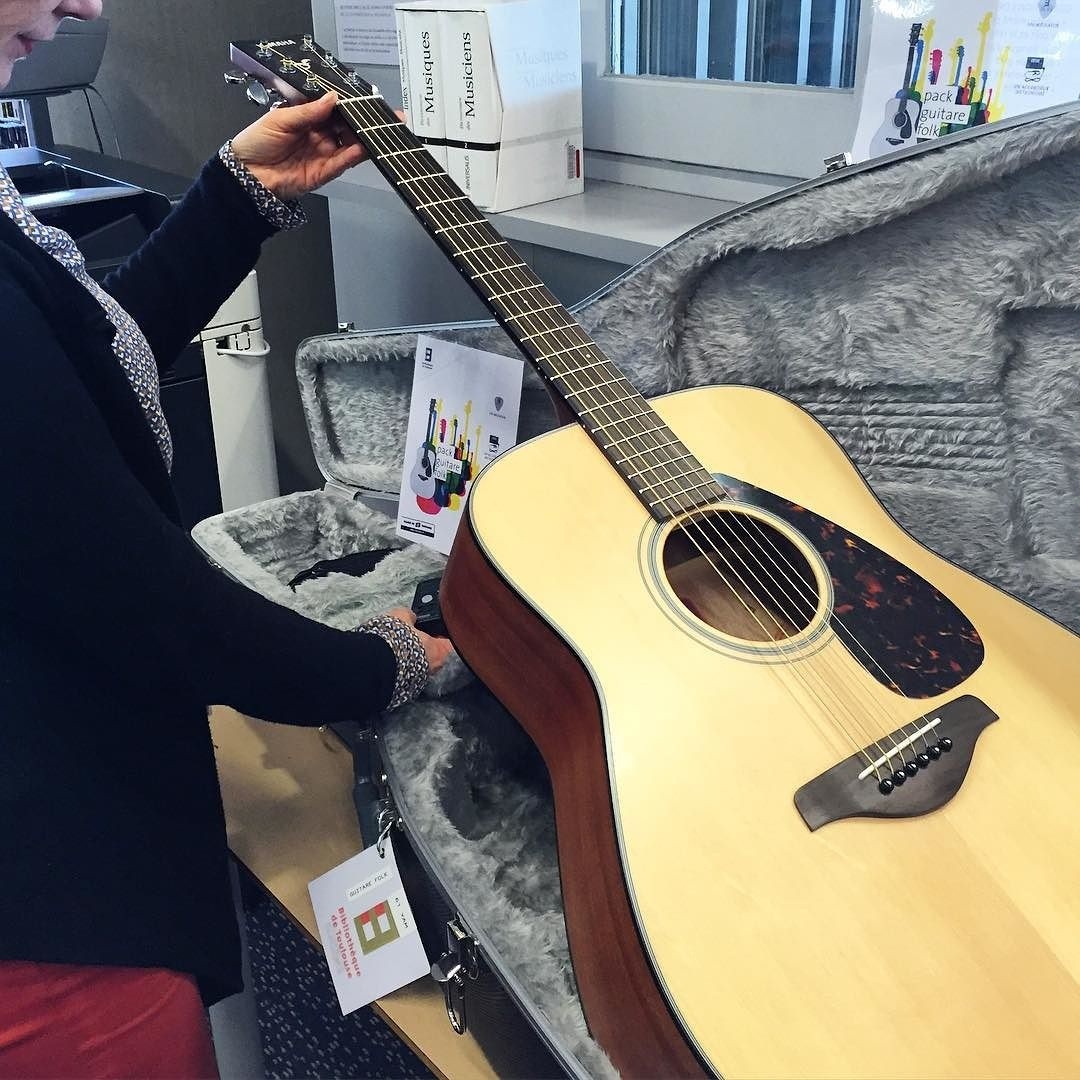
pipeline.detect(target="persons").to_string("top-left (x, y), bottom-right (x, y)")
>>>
top-left (0, 0), bottom-right (456, 1080)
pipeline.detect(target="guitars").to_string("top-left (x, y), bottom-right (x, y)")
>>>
top-left (219, 27), bottom-right (1077, 1079)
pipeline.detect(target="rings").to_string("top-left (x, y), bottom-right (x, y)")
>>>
top-left (336, 134), bottom-right (343, 148)
